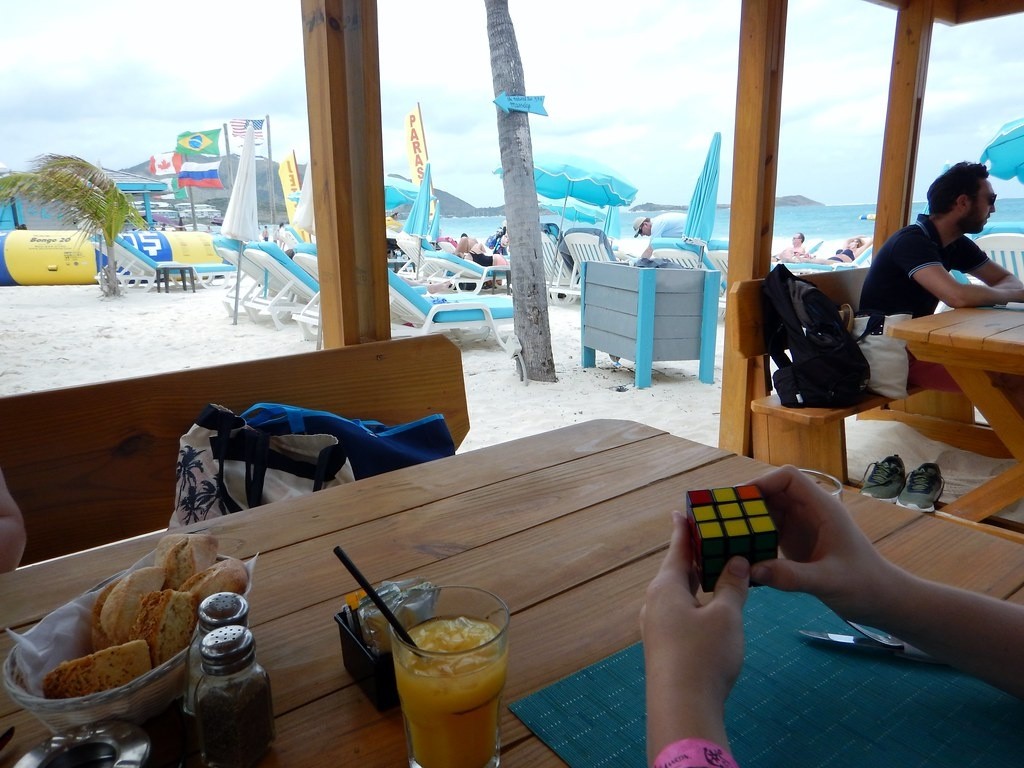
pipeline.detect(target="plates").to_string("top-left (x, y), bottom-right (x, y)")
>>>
top-left (11, 718), bottom-right (152, 767)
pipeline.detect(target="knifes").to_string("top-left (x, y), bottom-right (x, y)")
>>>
top-left (799, 629), bottom-right (939, 663)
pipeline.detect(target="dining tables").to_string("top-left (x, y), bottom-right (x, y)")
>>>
top-left (0, 418), bottom-right (1023, 768)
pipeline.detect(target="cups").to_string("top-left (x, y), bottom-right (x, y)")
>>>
top-left (800, 469), bottom-right (843, 503)
top-left (389, 585), bottom-right (512, 768)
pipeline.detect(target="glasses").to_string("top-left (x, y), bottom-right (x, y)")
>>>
top-left (991, 192), bottom-right (996, 205)
top-left (638, 226), bottom-right (642, 234)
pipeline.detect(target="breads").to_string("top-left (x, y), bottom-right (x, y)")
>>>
top-left (40, 533), bottom-right (248, 699)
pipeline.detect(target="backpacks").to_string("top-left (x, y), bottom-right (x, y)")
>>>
top-left (759, 264), bottom-right (870, 409)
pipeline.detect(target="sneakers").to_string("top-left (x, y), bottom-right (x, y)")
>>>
top-left (854, 453), bottom-right (945, 512)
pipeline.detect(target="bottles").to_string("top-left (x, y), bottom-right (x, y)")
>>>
top-left (194, 626), bottom-right (274, 767)
top-left (182, 591), bottom-right (248, 716)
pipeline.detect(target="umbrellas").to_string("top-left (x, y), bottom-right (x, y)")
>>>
top-left (491, 149), bottom-right (639, 308)
top-left (920, 159), bottom-right (952, 216)
top-left (680, 127), bottom-right (722, 268)
top-left (977, 116), bottom-right (1024, 186)
top-left (858, 212), bottom-right (876, 222)
top-left (219, 120), bottom-right (260, 327)
top-left (291, 160), bottom-right (441, 282)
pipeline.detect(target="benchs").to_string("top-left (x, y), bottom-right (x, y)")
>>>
top-left (728, 267), bottom-right (974, 484)
top-left (0, 334), bottom-right (470, 559)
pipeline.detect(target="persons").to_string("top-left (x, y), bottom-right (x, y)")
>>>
top-left (638, 462), bottom-right (1024, 768)
top-left (853, 160), bottom-right (1024, 419)
top-left (795, 234), bottom-right (874, 266)
top-left (274, 222), bottom-right (286, 251)
top-left (771, 232), bottom-right (805, 263)
top-left (395, 274), bottom-right (451, 295)
top-left (451, 232), bottom-right (510, 267)
top-left (262, 225), bottom-right (269, 242)
top-left (632, 212), bottom-right (688, 260)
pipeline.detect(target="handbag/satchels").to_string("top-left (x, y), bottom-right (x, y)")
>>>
top-left (169, 402), bottom-right (455, 528)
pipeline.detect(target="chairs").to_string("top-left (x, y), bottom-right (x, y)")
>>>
top-left (90, 223), bottom-right (1024, 351)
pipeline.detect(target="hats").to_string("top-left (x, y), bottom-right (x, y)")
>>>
top-left (633, 217), bottom-right (646, 238)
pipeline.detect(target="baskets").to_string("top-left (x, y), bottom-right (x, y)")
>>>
top-left (1, 553), bottom-right (250, 736)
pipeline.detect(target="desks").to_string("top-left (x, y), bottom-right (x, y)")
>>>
top-left (886, 309), bottom-right (1024, 522)
top-left (156, 264), bottom-right (196, 293)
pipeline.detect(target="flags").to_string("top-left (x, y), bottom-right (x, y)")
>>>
top-left (149, 176), bottom-right (189, 200)
top-left (229, 118), bottom-right (266, 149)
top-left (175, 127), bottom-right (223, 157)
top-left (178, 159), bottom-right (225, 190)
top-left (149, 151), bottom-right (184, 176)
